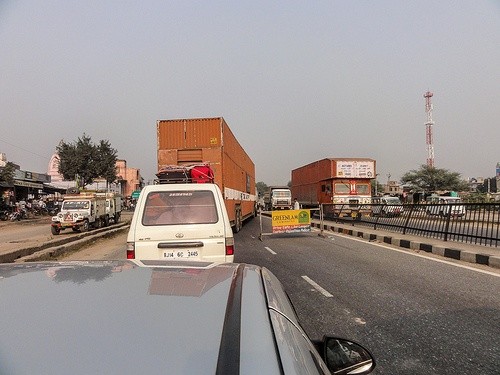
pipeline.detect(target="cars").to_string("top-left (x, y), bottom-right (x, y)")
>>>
top-left (1, 260), bottom-right (378, 375)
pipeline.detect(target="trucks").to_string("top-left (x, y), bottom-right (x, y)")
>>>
top-left (373, 195), bottom-right (405, 217)
top-left (157, 117), bottom-right (257, 232)
top-left (96, 192), bottom-right (122, 226)
top-left (50, 193), bottom-right (106, 235)
top-left (263, 185), bottom-right (293, 212)
top-left (292, 156), bottom-right (375, 220)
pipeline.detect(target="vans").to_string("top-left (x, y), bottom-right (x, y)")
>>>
top-left (126, 183), bottom-right (236, 263)
top-left (426, 195), bottom-right (467, 218)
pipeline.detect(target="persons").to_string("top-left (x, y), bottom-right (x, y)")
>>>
top-left (9, 197), bottom-right (27, 213)
top-left (38, 197), bottom-right (47, 215)
top-left (293, 198), bottom-right (299, 210)
